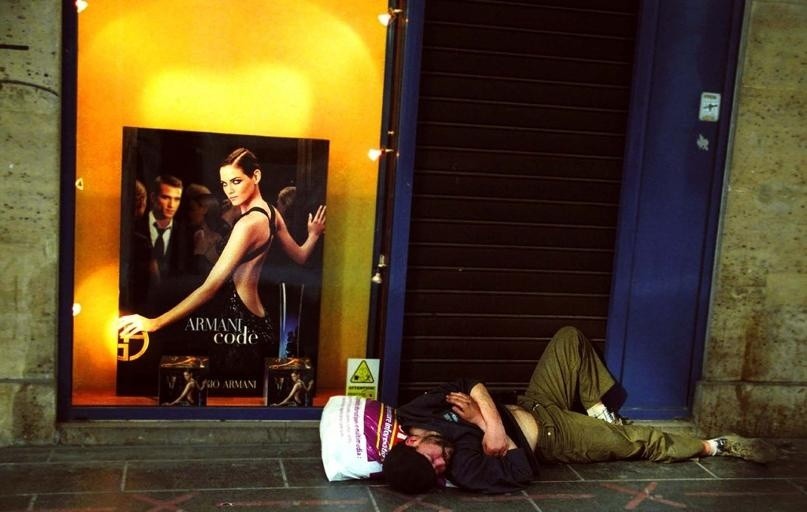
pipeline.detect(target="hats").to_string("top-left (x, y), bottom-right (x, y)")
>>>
top-left (383, 446), bottom-right (436, 496)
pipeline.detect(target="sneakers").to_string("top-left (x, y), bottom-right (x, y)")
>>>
top-left (716, 433), bottom-right (777, 464)
top-left (611, 419), bottom-right (624, 426)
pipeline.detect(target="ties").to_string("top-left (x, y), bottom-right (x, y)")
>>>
top-left (152, 222), bottom-right (172, 262)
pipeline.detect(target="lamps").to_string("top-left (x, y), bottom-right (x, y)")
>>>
top-left (367, 148), bottom-right (394, 161)
top-left (377, 9), bottom-right (402, 27)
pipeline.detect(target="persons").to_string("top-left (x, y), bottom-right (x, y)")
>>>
top-left (117, 148), bottom-right (325, 373)
top-left (128, 174), bottom-right (240, 338)
top-left (376, 324), bottom-right (780, 498)
top-left (277, 187), bottom-right (297, 224)
